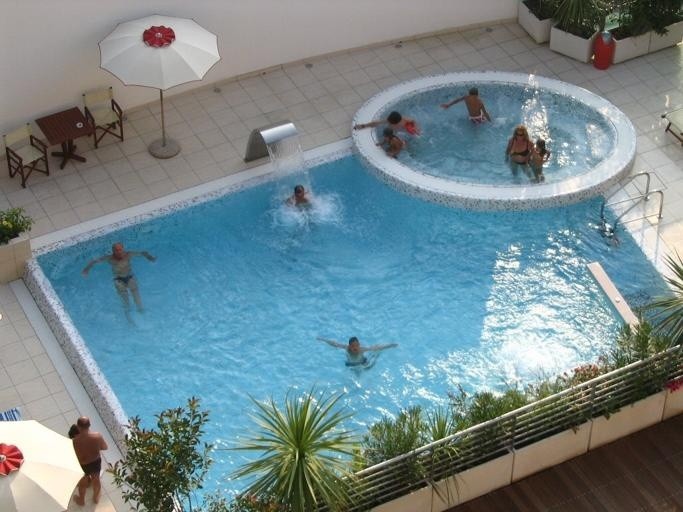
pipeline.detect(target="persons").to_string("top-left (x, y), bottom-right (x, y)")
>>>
top-left (439, 87), bottom-right (491, 125)
top-left (503, 124), bottom-right (533, 184)
top-left (81, 241), bottom-right (156, 324)
top-left (529, 140), bottom-right (550, 183)
top-left (375, 127), bottom-right (407, 157)
top-left (71, 415), bottom-right (107, 505)
top-left (283, 183), bottom-right (311, 209)
top-left (316, 336), bottom-right (399, 368)
top-left (352, 112), bottom-right (418, 135)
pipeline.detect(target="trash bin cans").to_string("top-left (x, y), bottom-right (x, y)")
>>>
top-left (593, 31), bottom-right (615, 70)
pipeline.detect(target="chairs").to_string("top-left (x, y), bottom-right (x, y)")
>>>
top-left (3, 123), bottom-right (49, 188)
top-left (661, 107), bottom-right (683, 146)
top-left (82, 87), bottom-right (124, 148)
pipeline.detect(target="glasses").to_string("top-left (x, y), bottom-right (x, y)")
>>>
top-left (517, 134), bottom-right (524, 136)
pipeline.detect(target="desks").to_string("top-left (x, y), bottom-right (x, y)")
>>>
top-left (35, 107), bottom-right (94, 169)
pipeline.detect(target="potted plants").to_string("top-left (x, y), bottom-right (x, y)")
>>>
top-left (0, 207), bottom-right (33, 285)
top-left (518, 0), bottom-right (683, 65)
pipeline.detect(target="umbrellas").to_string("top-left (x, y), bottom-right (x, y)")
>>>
top-left (96, 14), bottom-right (222, 145)
top-left (0, 419), bottom-right (85, 512)
